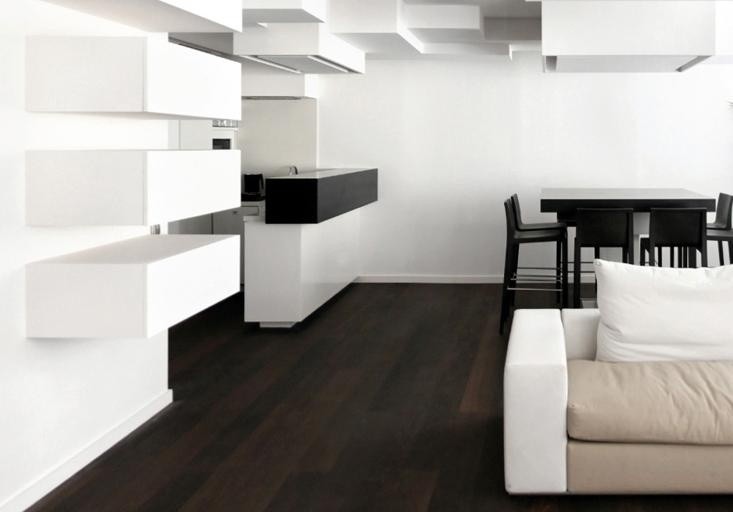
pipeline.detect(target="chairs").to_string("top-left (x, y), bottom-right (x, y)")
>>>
top-left (678, 193), bottom-right (733, 268)
top-left (573, 207), bottom-right (634, 308)
top-left (640, 207), bottom-right (707, 269)
top-left (499, 194), bottom-right (568, 334)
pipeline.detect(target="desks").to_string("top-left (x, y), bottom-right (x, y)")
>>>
top-left (540, 186), bottom-right (716, 265)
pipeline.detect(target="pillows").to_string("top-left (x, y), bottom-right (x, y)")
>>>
top-left (593, 257), bottom-right (733, 363)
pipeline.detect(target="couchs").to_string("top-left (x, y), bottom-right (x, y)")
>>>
top-left (504, 307), bottom-right (732, 507)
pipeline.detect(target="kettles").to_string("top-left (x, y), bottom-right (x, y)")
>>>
top-left (243, 171), bottom-right (265, 194)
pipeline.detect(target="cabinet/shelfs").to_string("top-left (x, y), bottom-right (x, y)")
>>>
top-left (25, 0), bottom-right (243, 340)
